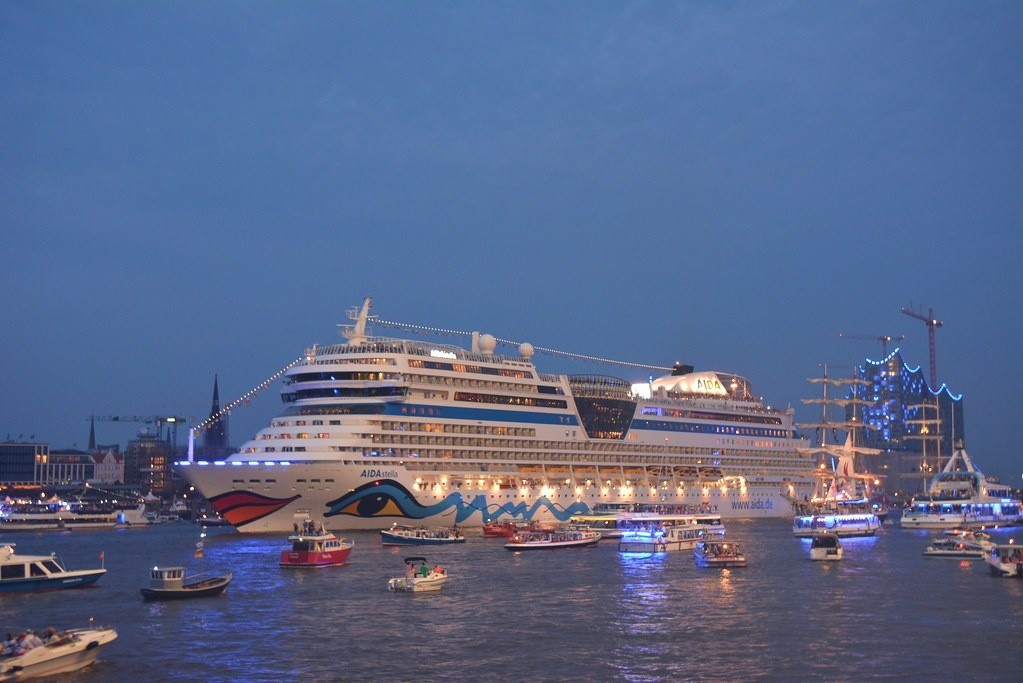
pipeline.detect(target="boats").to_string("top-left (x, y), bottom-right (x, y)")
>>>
top-left (380, 522), bottom-right (465, 547)
top-left (0, 542), bottom-right (108, 593)
top-left (899, 441), bottom-right (1023, 529)
top-left (566, 501), bottom-right (726, 554)
top-left (140, 567), bottom-right (233, 598)
top-left (388, 557), bottom-right (448, 592)
top-left (922, 524), bottom-right (1023, 579)
top-left (279, 508), bottom-right (356, 569)
top-left (503, 530), bottom-right (602, 551)
top-left (482, 518), bottom-right (557, 539)
top-left (0, 504), bottom-right (231, 533)
top-left (808, 534), bottom-right (844, 561)
top-left (694, 532), bottom-right (749, 569)
top-left (0, 616), bottom-right (118, 683)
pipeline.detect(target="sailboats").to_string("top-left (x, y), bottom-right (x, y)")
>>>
top-left (779, 362), bottom-right (890, 536)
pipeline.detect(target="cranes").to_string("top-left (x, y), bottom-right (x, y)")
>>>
top-left (900, 302), bottom-right (944, 392)
top-left (839, 333), bottom-right (905, 360)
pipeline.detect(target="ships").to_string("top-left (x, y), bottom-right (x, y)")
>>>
top-left (178, 295), bottom-right (827, 533)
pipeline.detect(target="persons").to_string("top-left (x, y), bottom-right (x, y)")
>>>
top-left (406, 561), bottom-right (443, 578)
top-left (0, 627), bottom-right (68, 656)
top-left (292, 519), bottom-right (315, 536)
top-left (400, 525), bottom-right (461, 541)
top-left (699, 545), bottom-right (736, 557)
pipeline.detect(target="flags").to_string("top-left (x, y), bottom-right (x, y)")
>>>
top-left (194, 539), bottom-right (203, 558)
top-left (97, 551), bottom-right (103, 561)
